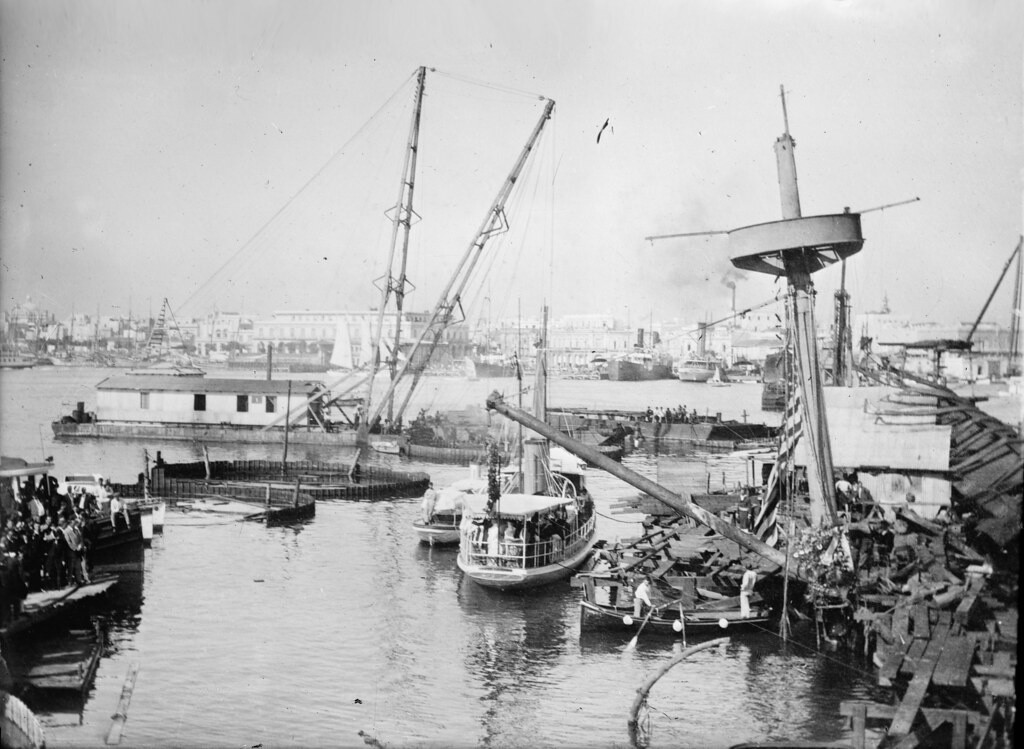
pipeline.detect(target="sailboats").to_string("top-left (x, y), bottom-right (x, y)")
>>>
top-left (125, 297), bottom-right (207, 377)
top-left (456, 305), bottom-right (598, 591)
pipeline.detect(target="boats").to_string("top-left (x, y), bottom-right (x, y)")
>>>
top-left (0, 455), bottom-right (167, 637)
top-left (578, 595), bottom-right (774, 638)
top-left (410, 459), bottom-right (486, 548)
top-left (325, 314), bottom-right (922, 416)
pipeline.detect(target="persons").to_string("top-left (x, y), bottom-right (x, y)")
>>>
top-left (0, 473), bottom-right (130, 625)
top-left (736, 488), bottom-right (752, 529)
top-left (324, 412), bottom-right (332, 432)
top-left (740, 563), bottom-right (757, 617)
top-left (353, 411), bottom-right (496, 448)
top-left (467, 489), bottom-right (592, 569)
top-left (634, 577), bottom-right (657, 618)
top-left (835, 479), bottom-right (874, 511)
top-left (646, 404), bottom-right (698, 424)
